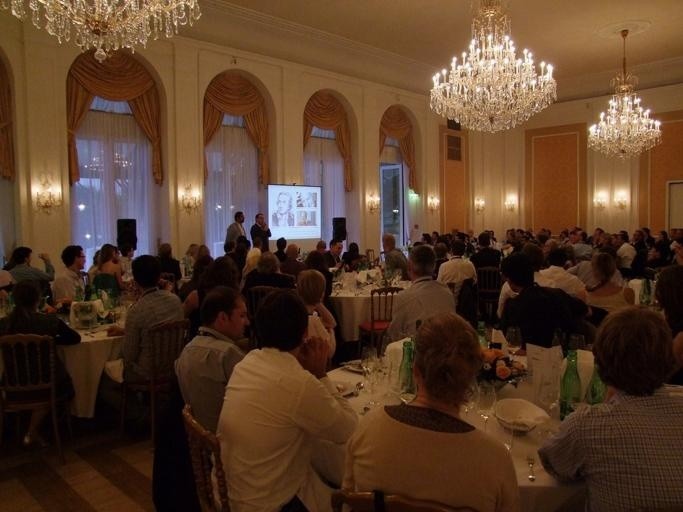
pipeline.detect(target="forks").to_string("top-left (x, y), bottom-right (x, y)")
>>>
top-left (526, 453), bottom-right (537, 480)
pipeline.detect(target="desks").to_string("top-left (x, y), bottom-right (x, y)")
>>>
top-left (309, 346), bottom-right (596, 508)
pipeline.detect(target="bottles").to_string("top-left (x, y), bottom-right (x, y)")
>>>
top-left (559, 350), bottom-right (580, 422)
top-left (584, 359), bottom-right (608, 404)
top-left (475, 321), bottom-right (528, 375)
top-left (637, 279), bottom-right (659, 312)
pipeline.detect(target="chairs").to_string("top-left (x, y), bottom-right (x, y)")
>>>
top-left (181, 403), bottom-right (229, 508)
top-left (1, 270), bottom-right (192, 454)
top-left (330, 489), bottom-right (460, 508)
top-left (252, 241), bottom-right (682, 360)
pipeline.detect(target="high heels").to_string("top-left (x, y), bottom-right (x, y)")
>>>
top-left (23, 430), bottom-right (51, 449)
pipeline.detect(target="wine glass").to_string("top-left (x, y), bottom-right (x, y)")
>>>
top-left (360, 336), bottom-right (416, 408)
top-left (465, 381), bottom-right (552, 469)
top-left (330, 269), bottom-right (402, 297)
top-left (0, 269), bottom-right (130, 340)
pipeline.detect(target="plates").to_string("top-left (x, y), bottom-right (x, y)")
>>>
top-left (343, 359), bottom-right (362, 371)
top-left (330, 382), bottom-right (352, 396)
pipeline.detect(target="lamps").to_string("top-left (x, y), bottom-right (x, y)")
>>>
top-left (425, 1), bottom-right (559, 137)
top-left (178, 192), bottom-right (203, 217)
top-left (367, 195), bottom-right (380, 214)
top-left (425, 201), bottom-right (439, 213)
top-left (474, 202), bottom-right (485, 215)
top-left (614, 199), bottom-right (628, 209)
top-left (32, 183), bottom-right (64, 216)
top-left (502, 201), bottom-right (518, 212)
top-left (591, 198), bottom-right (606, 210)
top-left (580, 27), bottom-right (665, 162)
top-left (0, 0), bottom-right (206, 64)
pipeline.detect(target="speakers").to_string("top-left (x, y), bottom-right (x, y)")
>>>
top-left (116, 218), bottom-right (137, 254)
top-left (333, 217), bottom-right (347, 241)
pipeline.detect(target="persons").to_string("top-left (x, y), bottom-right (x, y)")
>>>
top-left (272, 192), bottom-right (293, 226)
